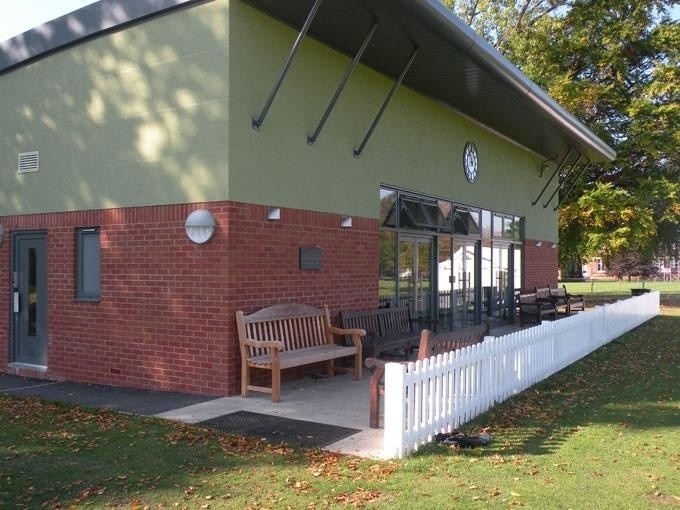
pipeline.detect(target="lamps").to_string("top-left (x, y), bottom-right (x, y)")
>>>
top-left (183, 209), bottom-right (216, 245)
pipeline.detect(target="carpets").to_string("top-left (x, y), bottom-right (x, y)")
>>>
top-left (190, 409), bottom-right (360, 451)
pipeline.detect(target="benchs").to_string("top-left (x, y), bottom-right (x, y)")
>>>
top-left (363, 319), bottom-right (491, 431)
top-left (236, 301), bottom-right (366, 402)
top-left (338, 304), bottom-right (426, 364)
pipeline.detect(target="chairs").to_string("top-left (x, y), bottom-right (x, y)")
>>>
top-left (513, 284), bottom-right (586, 327)
